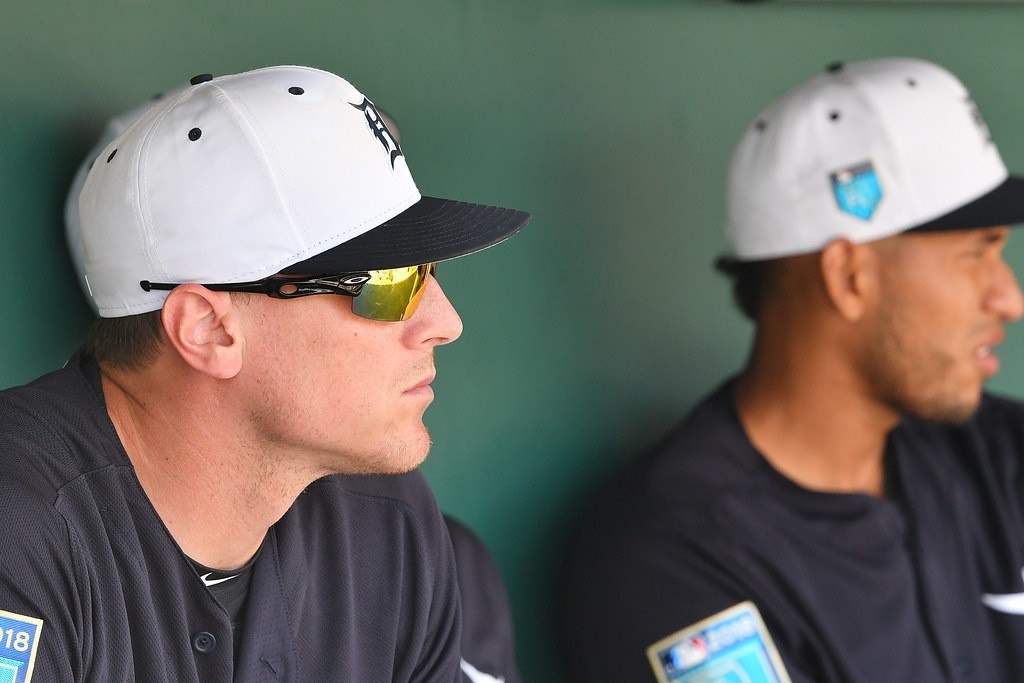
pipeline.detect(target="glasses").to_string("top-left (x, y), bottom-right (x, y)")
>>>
top-left (140, 261), bottom-right (438, 321)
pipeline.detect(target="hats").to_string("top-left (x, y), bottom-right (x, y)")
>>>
top-left (725, 56), bottom-right (1023, 265)
top-left (65, 63), bottom-right (533, 319)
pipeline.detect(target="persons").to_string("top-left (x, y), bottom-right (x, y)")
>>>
top-left (0, 63), bottom-right (529, 683)
top-left (560, 56), bottom-right (1024, 683)
